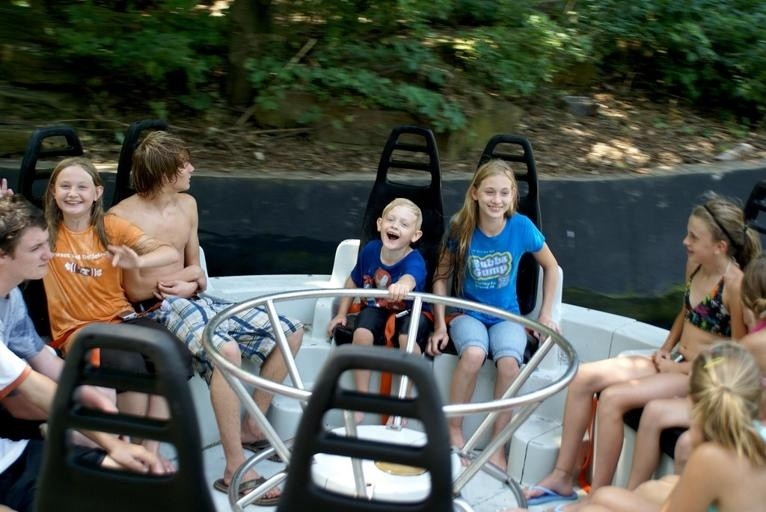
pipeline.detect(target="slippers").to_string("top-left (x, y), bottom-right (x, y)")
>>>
top-left (242, 440), bottom-right (291, 461)
top-left (215, 476), bottom-right (280, 506)
top-left (526, 482), bottom-right (578, 512)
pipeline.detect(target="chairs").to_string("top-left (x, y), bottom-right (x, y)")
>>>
top-left (14, 125), bottom-right (83, 337)
top-left (109, 120), bottom-right (170, 207)
top-left (597, 178), bottom-right (765, 458)
top-left (31, 321), bottom-right (216, 512)
top-left (277, 349), bottom-right (452, 512)
top-left (334, 128), bottom-right (444, 349)
top-left (434, 134), bottom-right (543, 364)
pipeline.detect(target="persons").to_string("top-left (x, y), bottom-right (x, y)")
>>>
top-left (0, 190), bottom-right (120, 448)
top-left (522, 199), bottom-right (766, 511)
top-left (424, 160), bottom-right (558, 476)
top-left (0, 341), bottom-right (170, 512)
top-left (328, 196), bottom-right (429, 428)
top-left (106, 130), bottom-right (305, 506)
top-left (0, 157), bottom-right (179, 450)
